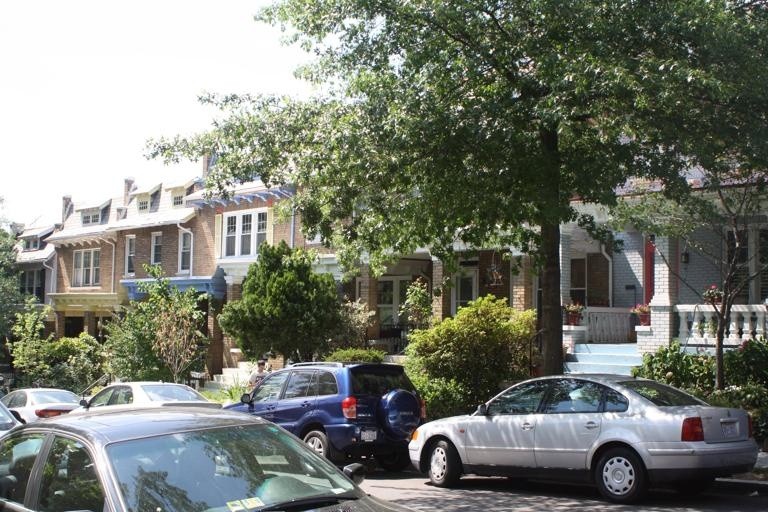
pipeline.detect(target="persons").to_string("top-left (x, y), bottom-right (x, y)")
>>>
top-left (246, 359), bottom-right (270, 385)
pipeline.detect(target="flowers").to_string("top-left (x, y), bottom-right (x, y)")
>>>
top-left (562, 300), bottom-right (584, 314)
top-left (702, 283), bottom-right (721, 298)
top-left (630, 303), bottom-right (651, 314)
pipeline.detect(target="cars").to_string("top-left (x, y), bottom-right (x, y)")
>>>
top-left (408, 374), bottom-right (758, 503)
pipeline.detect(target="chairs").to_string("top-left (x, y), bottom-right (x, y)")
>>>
top-left (175, 445), bottom-right (225, 509)
top-left (551, 393), bottom-right (592, 411)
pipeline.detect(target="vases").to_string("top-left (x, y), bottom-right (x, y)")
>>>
top-left (566, 312), bottom-right (581, 325)
top-left (703, 297), bottom-right (721, 305)
top-left (637, 313), bottom-right (650, 326)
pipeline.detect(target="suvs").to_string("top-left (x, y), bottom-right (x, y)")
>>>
top-left (221, 362), bottom-right (427, 475)
